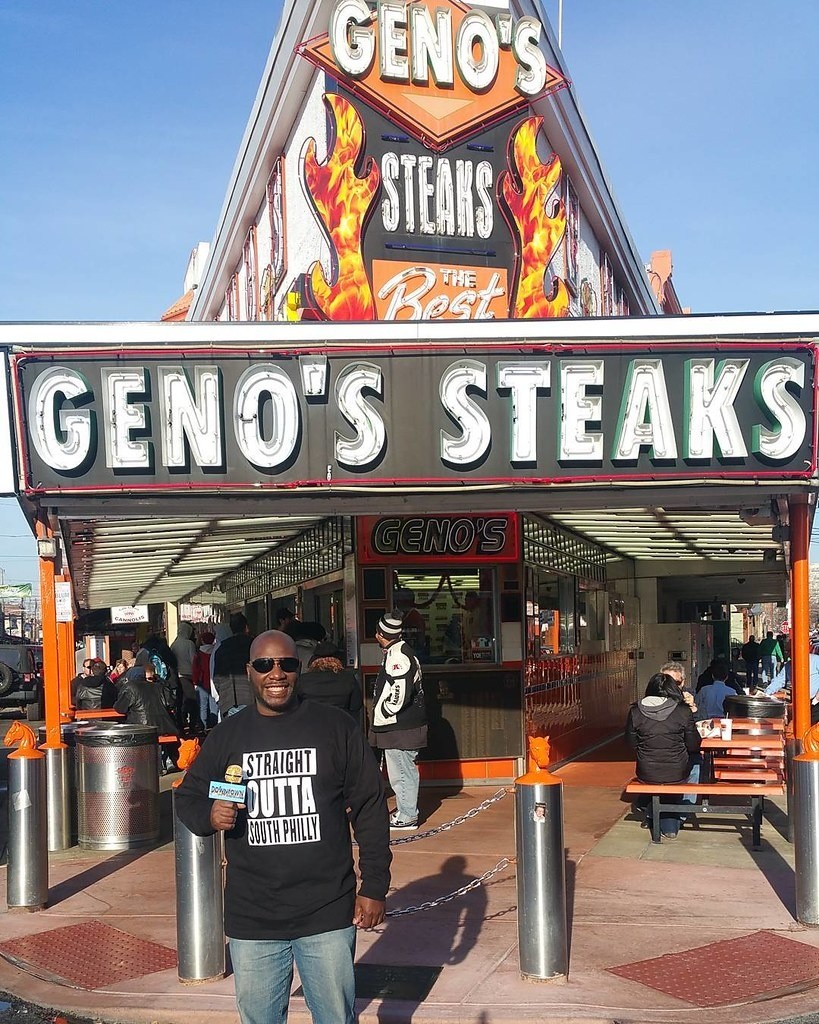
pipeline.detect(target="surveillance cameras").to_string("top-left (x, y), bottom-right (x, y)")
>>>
top-left (746, 507), bottom-right (760, 517)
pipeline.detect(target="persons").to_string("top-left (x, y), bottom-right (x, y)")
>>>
top-left (368, 614), bottom-right (429, 830)
top-left (174, 629), bottom-right (392, 1024)
top-left (623, 631), bottom-right (819, 841)
top-left (462, 592), bottom-right (490, 659)
top-left (394, 589), bottom-right (426, 664)
top-left (70, 608), bottom-right (371, 776)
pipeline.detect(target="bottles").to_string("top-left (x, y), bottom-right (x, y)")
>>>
top-left (402, 624), bottom-right (419, 632)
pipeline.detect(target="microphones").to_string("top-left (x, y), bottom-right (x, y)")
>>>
top-left (209, 764), bottom-right (247, 803)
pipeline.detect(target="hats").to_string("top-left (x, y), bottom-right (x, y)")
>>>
top-left (376, 608), bottom-right (403, 639)
top-left (308, 642), bottom-right (344, 667)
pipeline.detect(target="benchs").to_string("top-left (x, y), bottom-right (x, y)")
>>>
top-left (626, 751), bottom-right (786, 847)
top-left (157, 735), bottom-right (177, 776)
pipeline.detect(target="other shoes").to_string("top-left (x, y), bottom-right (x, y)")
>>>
top-left (641, 818), bottom-right (652, 829)
top-left (662, 833), bottom-right (677, 840)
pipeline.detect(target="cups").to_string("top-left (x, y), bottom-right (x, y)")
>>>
top-left (743, 688), bottom-right (749, 695)
top-left (720, 719), bottom-right (733, 740)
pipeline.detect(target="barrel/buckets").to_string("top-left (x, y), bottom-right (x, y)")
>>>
top-left (401, 632), bottom-right (418, 650)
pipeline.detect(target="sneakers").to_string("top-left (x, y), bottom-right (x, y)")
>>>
top-left (390, 815), bottom-right (419, 830)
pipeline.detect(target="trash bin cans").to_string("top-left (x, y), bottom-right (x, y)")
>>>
top-left (39, 725), bottom-right (160, 852)
top-left (722, 695), bottom-right (786, 735)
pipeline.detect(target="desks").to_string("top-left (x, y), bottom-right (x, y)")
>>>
top-left (75, 708), bottom-right (127, 718)
top-left (711, 718), bottom-right (785, 734)
top-left (703, 733), bottom-right (784, 784)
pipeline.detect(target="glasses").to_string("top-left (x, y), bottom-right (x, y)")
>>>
top-left (85, 666), bottom-right (91, 669)
top-left (677, 679), bottom-right (683, 686)
top-left (252, 657), bottom-right (299, 673)
